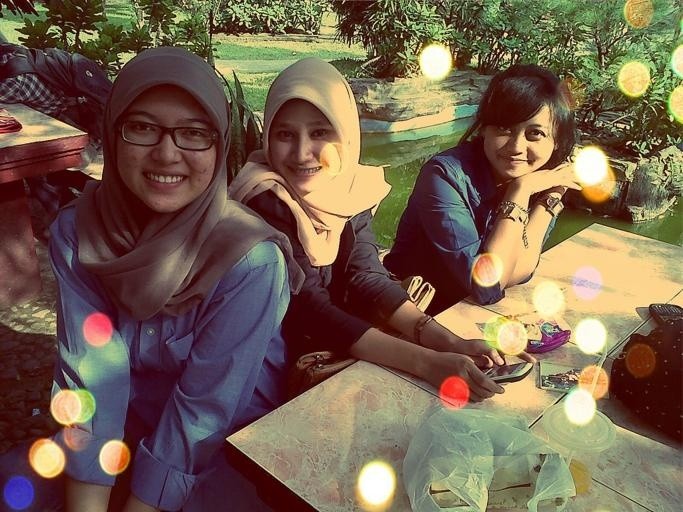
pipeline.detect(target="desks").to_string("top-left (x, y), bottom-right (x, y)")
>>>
top-left (0, 99), bottom-right (92, 309)
top-left (224, 221), bottom-right (682, 511)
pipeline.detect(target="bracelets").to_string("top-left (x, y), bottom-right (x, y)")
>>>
top-left (414, 315), bottom-right (433, 346)
top-left (496, 200), bottom-right (531, 225)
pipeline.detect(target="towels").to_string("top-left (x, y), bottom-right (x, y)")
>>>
top-left (1, 106), bottom-right (22, 134)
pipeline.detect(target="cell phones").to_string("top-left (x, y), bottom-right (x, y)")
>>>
top-left (481, 362), bottom-right (533, 383)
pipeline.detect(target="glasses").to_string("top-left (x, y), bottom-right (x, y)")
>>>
top-left (117, 120), bottom-right (216, 151)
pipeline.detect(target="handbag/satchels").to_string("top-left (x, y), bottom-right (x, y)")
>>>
top-left (295, 274), bottom-right (437, 390)
top-left (609, 319), bottom-right (683, 443)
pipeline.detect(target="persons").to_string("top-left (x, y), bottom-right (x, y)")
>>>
top-left (228, 57), bottom-right (537, 404)
top-left (0, 47), bottom-right (305, 512)
top-left (0, 43), bottom-right (113, 238)
top-left (383, 63), bottom-right (584, 317)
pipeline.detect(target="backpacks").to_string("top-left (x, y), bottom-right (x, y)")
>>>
top-left (2, 42), bottom-right (110, 118)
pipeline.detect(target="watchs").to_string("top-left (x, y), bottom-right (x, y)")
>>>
top-left (536, 193), bottom-right (564, 216)
